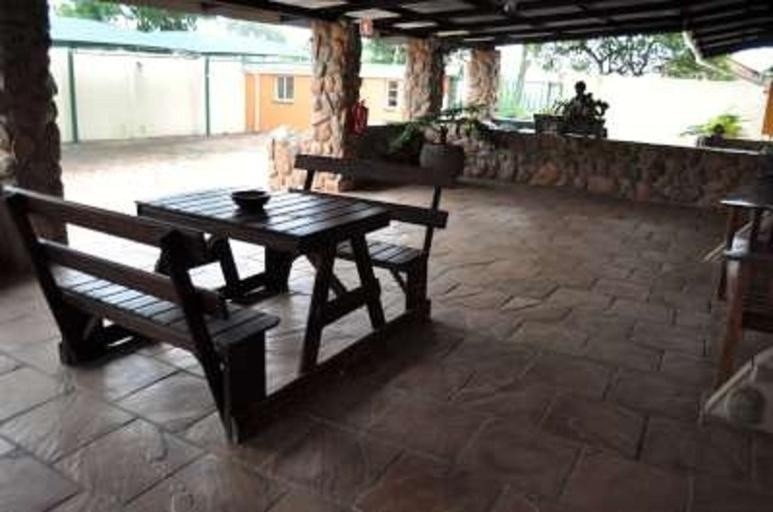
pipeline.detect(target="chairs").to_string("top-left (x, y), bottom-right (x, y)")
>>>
top-left (705, 197), bottom-right (772, 384)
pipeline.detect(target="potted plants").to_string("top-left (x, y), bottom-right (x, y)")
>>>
top-left (384, 107), bottom-right (490, 177)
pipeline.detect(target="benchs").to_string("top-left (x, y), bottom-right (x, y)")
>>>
top-left (288, 153), bottom-right (447, 312)
top-left (1, 182), bottom-right (280, 446)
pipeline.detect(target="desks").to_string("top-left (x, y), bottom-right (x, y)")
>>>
top-left (129, 184), bottom-right (387, 371)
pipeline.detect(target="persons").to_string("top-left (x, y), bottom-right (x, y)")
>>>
top-left (562, 81), bottom-right (594, 114)
